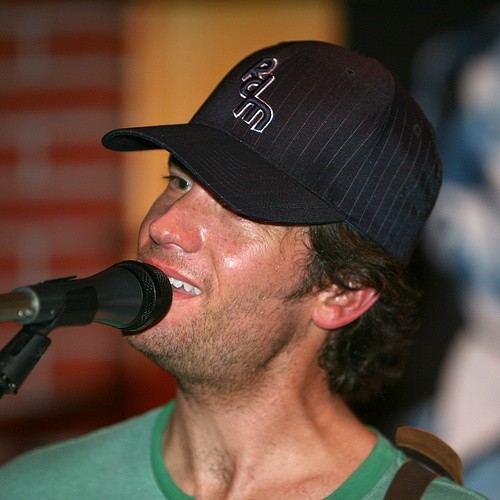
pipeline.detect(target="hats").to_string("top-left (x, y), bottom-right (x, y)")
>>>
top-left (102, 40), bottom-right (445, 262)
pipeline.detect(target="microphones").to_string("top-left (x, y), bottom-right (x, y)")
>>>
top-left (0, 260), bottom-right (174, 336)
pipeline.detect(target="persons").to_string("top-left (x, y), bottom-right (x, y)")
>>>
top-left (0, 40), bottom-right (492, 500)
